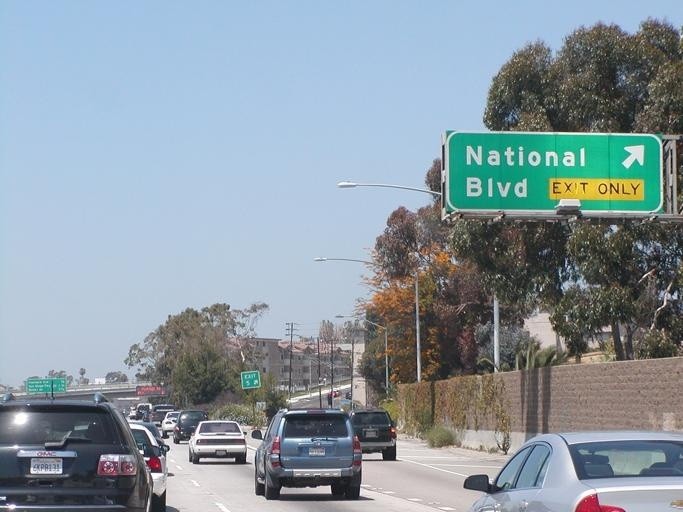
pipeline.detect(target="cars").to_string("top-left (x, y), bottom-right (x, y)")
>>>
top-left (128, 402), bottom-right (180, 438)
top-left (461, 429), bottom-right (683, 512)
top-left (188, 420), bottom-right (248, 464)
top-left (128, 420), bottom-right (170, 511)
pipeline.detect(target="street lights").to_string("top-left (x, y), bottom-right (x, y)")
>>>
top-left (314, 256), bottom-right (421, 382)
top-left (338, 181), bottom-right (502, 374)
top-left (333, 312), bottom-right (389, 397)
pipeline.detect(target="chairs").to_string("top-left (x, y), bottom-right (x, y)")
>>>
top-left (583, 455), bottom-right (615, 478)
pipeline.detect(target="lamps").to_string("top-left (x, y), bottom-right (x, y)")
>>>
top-left (555, 198), bottom-right (581, 214)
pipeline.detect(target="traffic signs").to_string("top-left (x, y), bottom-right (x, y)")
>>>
top-left (28, 376), bottom-right (67, 394)
top-left (441, 130), bottom-right (664, 224)
top-left (241, 371), bottom-right (260, 390)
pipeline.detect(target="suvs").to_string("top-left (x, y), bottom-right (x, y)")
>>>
top-left (172, 409), bottom-right (208, 442)
top-left (250, 403), bottom-right (362, 499)
top-left (1, 392), bottom-right (154, 512)
top-left (348, 410), bottom-right (397, 461)
